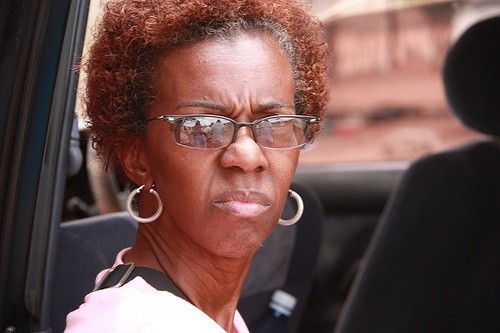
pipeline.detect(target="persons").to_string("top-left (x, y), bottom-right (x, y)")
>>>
top-left (60, 0), bottom-right (332, 333)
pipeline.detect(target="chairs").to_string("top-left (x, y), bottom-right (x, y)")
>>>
top-left (331, 15), bottom-right (500, 333)
top-left (49, 176), bottom-right (325, 333)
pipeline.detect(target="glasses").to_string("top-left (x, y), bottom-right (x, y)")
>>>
top-left (141, 114), bottom-right (320, 150)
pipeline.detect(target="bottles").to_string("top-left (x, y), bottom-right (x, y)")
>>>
top-left (252, 289), bottom-right (296, 333)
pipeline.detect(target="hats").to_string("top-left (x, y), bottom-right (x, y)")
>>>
top-left (443, 16), bottom-right (500, 136)
